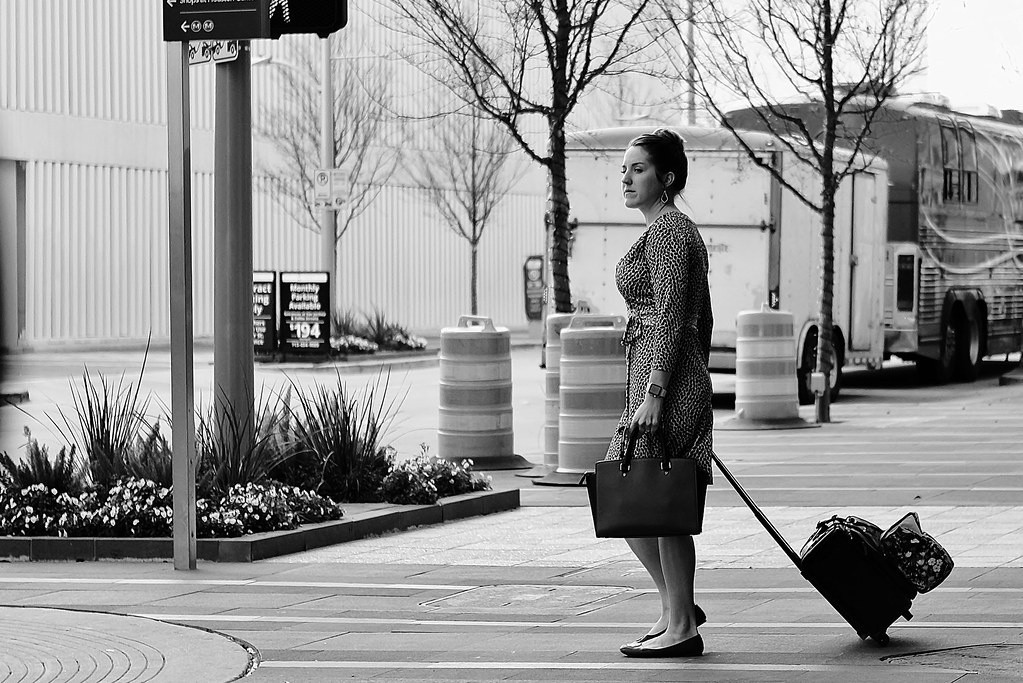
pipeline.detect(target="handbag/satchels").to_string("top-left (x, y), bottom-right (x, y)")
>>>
top-left (879, 511), bottom-right (954, 594)
top-left (578, 424), bottom-right (706, 538)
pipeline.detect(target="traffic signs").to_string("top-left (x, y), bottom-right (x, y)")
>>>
top-left (162, 0), bottom-right (272, 43)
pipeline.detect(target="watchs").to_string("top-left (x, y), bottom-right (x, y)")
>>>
top-left (646, 383), bottom-right (667, 397)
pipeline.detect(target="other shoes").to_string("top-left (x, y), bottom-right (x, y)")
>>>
top-left (620, 634), bottom-right (704, 658)
top-left (636, 604), bottom-right (706, 645)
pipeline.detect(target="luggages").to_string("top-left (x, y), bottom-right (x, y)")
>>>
top-left (711, 452), bottom-right (918, 642)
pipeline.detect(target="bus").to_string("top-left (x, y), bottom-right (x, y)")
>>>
top-left (718, 80), bottom-right (1022, 386)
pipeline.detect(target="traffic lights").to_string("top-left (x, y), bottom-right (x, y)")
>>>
top-left (271, 0), bottom-right (348, 41)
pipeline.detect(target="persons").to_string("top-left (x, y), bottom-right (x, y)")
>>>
top-left (603, 130), bottom-right (713, 658)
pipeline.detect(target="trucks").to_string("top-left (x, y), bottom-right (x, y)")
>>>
top-left (540, 123), bottom-right (885, 408)
